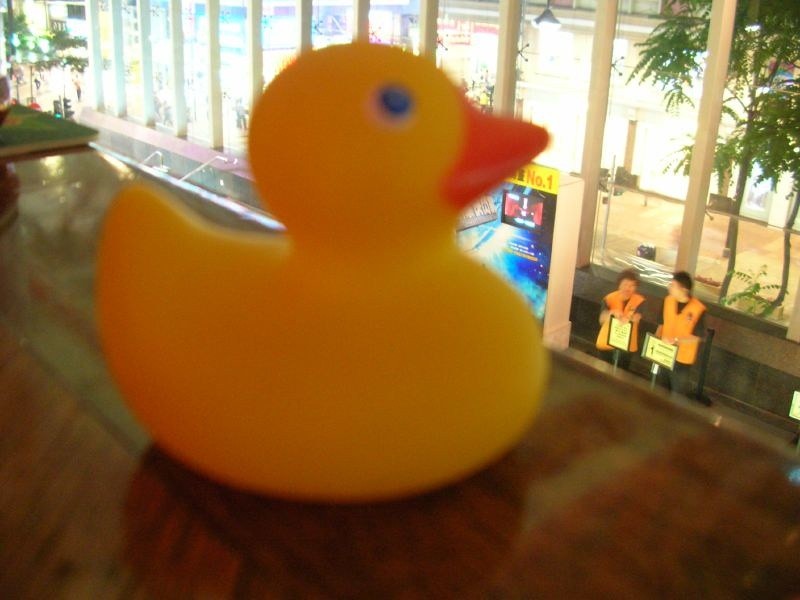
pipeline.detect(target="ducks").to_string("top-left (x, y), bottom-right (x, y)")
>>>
top-left (93, 45), bottom-right (548, 498)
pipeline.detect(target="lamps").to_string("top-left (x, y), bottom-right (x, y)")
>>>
top-left (532, 0), bottom-right (561, 25)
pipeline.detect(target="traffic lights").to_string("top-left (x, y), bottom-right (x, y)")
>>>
top-left (62, 97), bottom-right (74, 119)
top-left (54, 100), bottom-right (63, 119)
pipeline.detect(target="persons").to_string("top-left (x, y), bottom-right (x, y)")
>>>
top-left (655, 271), bottom-right (707, 396)
top-left (595, 269), bottom-right (646, 370)
